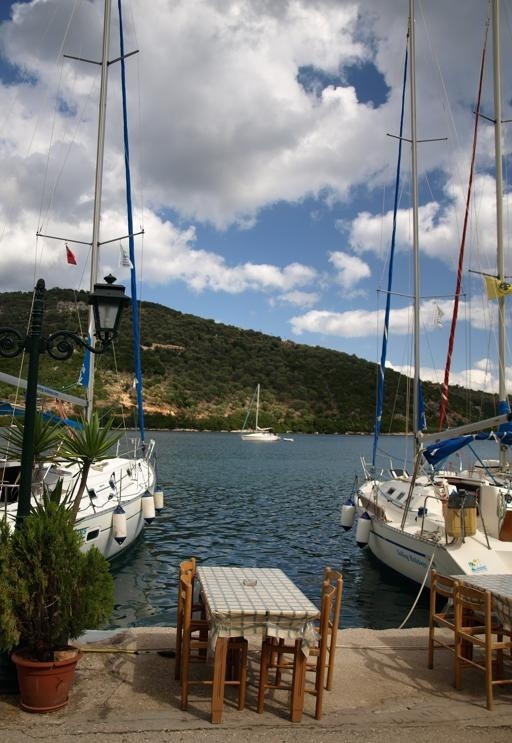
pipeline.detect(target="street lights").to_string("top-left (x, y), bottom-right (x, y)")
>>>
top-left (2, 274), bottom-right (131, 538)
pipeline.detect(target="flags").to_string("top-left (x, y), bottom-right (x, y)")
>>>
top-left (65, 246), bottom-right (77, 266)
top-left (481, 274), bottom-right (511, 300)
top-left (118, 244), bottom-right (136, 271)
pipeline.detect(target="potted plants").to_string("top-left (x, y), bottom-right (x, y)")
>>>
top-left (0, 405), bottom-right (125, 716)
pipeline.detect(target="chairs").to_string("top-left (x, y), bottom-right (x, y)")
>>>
top-left (425, 565), bottom-right (512, 709)
top-left (173, 554), bottom-right (347, 724)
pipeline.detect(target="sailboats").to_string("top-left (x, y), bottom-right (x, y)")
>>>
top-left (338, 0), bottom-right (510, 598)
top-left (0, 1), bottom-right (163, 562)
top-left (240, 383), bottom-right (280, 442)
